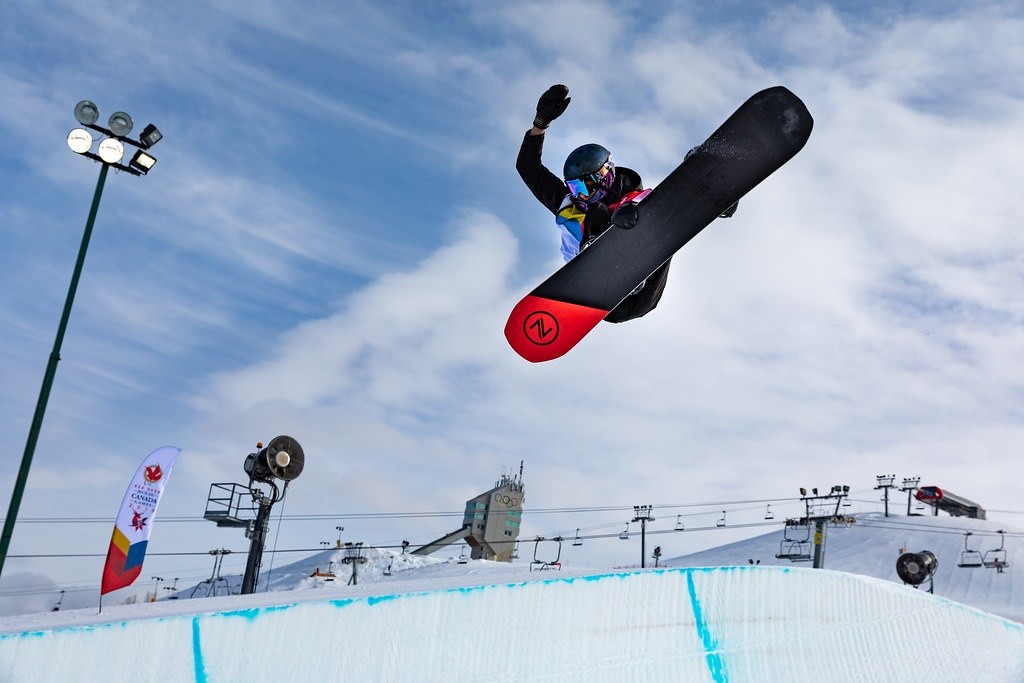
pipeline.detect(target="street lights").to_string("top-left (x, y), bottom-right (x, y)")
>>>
top-left (0, 99), bottom-right (166, 577)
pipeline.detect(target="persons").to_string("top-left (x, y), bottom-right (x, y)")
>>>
top-left (516, 85), bottom-right (739, 324)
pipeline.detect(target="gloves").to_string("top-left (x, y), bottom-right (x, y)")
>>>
top-left (611, 202), bottom-right (639, 230)
top-left (532, 84), bottom-right (571, 130)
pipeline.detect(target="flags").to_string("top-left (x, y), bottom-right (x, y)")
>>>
top-left (101, 445), bottom-right (181, 591)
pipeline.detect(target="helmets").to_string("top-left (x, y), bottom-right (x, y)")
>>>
top-left (564, 143), bottom-right (611, 180)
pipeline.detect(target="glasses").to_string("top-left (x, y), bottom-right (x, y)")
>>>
top-left (563, 173), bottom-right (598, 198)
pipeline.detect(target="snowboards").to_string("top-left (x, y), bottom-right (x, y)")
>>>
top-left (504, 86), bottom-right (813, 363)
